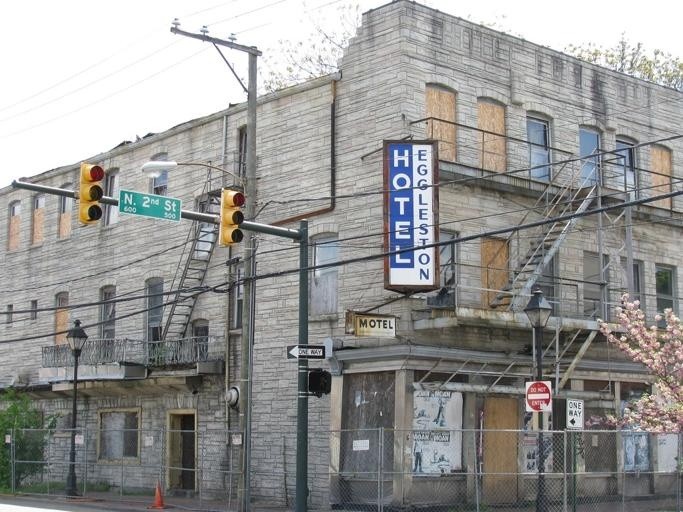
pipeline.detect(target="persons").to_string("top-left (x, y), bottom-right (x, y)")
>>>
top-left (413, 440), bottom-right (423, 472)
top-left (435, 398), bottom-right (448, 427)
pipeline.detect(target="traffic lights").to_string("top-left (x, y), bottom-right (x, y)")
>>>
top-left (215, 189), bottom-right (244, 247)
top-left (77, 163), bottom-right (104, 227)
top-left (308, 370), bottom-right (332, 396)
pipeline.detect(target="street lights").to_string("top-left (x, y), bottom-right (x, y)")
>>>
top-left (65, 318), bottom-right (88, 496)
top-left (142, 161), bottom-right (252, 512)
top-left (523, 288), bottom-right (555, 512)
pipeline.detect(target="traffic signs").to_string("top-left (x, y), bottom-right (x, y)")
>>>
top-left (565, 400), bottom-right (585, 429)
top-left (117, 189), bottom-right (181, 225)
top-left (284, 346), bottom-right (326, 358)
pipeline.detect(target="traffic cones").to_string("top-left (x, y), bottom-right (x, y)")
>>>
top-left (148, 477), bottom-right (165, 508)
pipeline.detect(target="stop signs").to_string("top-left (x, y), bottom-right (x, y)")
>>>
top-left (523, 380), bottom-right (552, 412)
